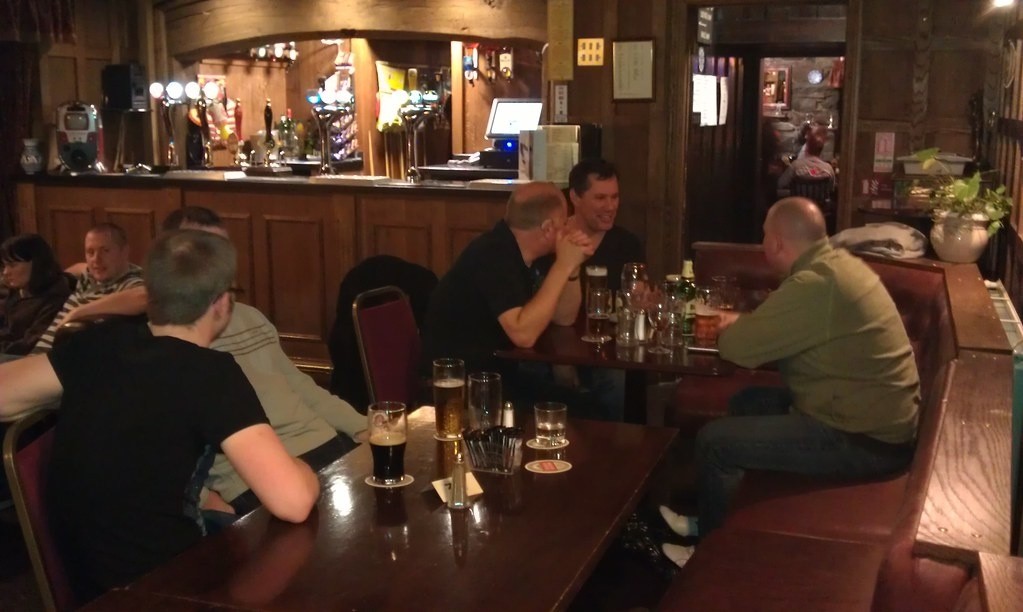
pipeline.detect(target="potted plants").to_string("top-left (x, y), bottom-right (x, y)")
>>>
top-left (894, 144), bottom-right (1013, 263)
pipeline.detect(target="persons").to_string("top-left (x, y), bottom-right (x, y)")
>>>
top-left (761, 123), bottom-right (838, 204)
top-left (0, 225), bottom-right (319, 596)
top-left (28, 222), bottom-right (151, 355)
top-left (420, 179), bottom-right (598, 414)
top-left (1, 234), bottom-right (72, 358)
top-left (657, 196), bottom-right (923, 570)
top-left (163, 206), bottom-right (372, 518)
top-left (537, 156), bottom-right (646, 421)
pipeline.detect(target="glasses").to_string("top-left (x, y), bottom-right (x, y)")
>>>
top-left (212, 288), bottom-right (245, 304)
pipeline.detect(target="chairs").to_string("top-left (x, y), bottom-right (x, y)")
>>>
top-left (0, 405), bottom-right (81, 612)
top-left (792, 176), bottom-right (833, 221)
top-left (351, 286), bottom-right (421, 412)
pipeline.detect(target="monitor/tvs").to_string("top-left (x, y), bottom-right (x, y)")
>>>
top-left (484, 98), bottom-right (544, 151)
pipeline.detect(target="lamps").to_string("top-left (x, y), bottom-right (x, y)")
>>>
top-left (149, 80), bottom-right (221, 171)
top-left (248, 43), bottom-right (299, 67)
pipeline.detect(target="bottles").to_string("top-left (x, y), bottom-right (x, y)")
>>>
top-left (677, 259), bottom-right (698, 337)
top-left (279, 110), bottom-right (300, 161)
top-left (634, 308), bottom-right (645, 342)
top-left (781, 81), bottom-right (786, 104)
top-left (20, 139), bottom-right (43, 175)
top-left (304, 120), bottom-right (315, 154)
top-left (504, 402), bottom-right (514, 427)
top-left (296, 120), bottom-right (304, 154)
top-left (765, 83), bottom-right (772, 103)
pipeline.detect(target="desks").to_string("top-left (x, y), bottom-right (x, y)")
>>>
top-left (858, 201), bottom-right (932, 230)
top-left (83, 401), bottom-right (685, 612)
top-left (495, 302), bottom-right (732, 424)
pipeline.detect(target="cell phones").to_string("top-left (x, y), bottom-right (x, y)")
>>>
top-left (688, 345), bottom-right (720, 353)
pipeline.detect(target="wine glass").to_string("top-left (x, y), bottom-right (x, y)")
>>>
top-left (623, 263), bottom-right (648, 345)
top-left (648, 280), bottom-right (673, 355)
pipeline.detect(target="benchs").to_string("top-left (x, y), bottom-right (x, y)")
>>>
top-left (646, 236), bottom-right (1023, 612)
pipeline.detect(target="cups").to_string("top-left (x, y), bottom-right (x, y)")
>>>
top-left (589, 288), bottom-right (612, 339)
top-left (662, 296), bottom-right (686, 347)
top-left (368, 401), bottom-right (406, 485)
top-left (710, 275), bottom-right (736, 309)
top-left (694, 287), bottom-right (719, 349)
top-left (616, 289), bottom-right (640, 346)
top-left (586, 266), bottom-right (608, 314)
top-left (533, 403), bottom-right (567, 447)
top-left (467, 372), bottom-right (502, 430)
top-left (433, 358), bottom-right (466, 439)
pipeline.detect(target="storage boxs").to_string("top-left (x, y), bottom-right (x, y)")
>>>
top-left (896, 156), bottom-right (973, 176)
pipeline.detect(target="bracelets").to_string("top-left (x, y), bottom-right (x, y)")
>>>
top-left (568, 274), bottom-right (580, 281)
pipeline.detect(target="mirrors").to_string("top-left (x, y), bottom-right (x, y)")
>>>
top-left (762, 66), bottom-right (792, 110)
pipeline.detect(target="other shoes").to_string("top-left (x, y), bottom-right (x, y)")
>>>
top-left (662, 543), bottom-right (696, 569)
top-left (657, 505), bottom-right (699, 538)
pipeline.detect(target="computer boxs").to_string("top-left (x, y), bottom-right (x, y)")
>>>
top-left (479, 151), bottom-right (519, 170)
